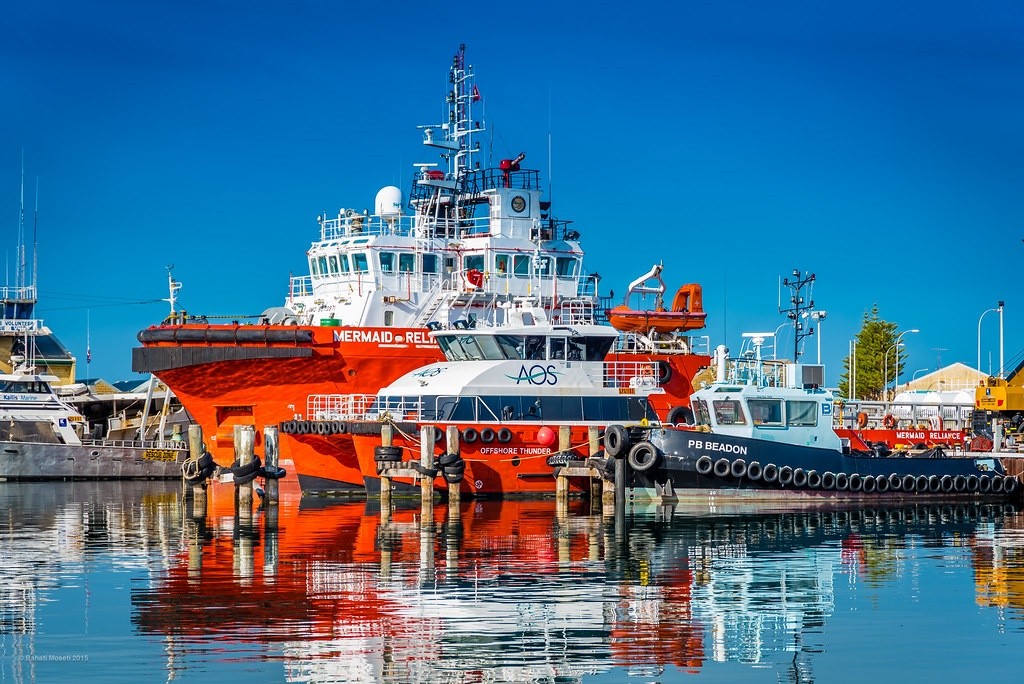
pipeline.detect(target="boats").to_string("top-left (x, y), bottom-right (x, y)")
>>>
top-left (130, 43), bottom-right (1024, 515)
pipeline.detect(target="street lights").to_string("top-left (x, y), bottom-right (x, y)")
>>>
top-left (913, 368), bottom-right (929, 394)
top-left (895, 329), bottom-right (920, 396)
top-left (884, 344), bottom-right (905, 400)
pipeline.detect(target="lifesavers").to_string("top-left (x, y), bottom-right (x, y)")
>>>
top-left (284, 419), bottom-right (346, 436)
top-left (882, 414), bottom-right (895, 428)
top-left (467, 269), bottom-right (483, 287)
top-left (374, 446), bottom-right (403, 462)
top-left (230, 454), bottom-right (288, 485)
top-left (435, 427), bottom-right (443, 443)
top-left (546, 454), bottom-right (580, 466)
top-left (667, 405), bottom-right (695, 428)
top-left (416, 451), bottom-right (465, 484)
top-left (696, 456), bottom-right (1020, 492)
top-left (628, 442), bottom-right (659, 471)
top-left (183, 452), bottom-right (213, 485)
top-left (603, 424), bottom-right (629, 457)
top-left (650, 360), bottom-right (672, 383)
top-left (463, 427), bottom-right (512, 444)
